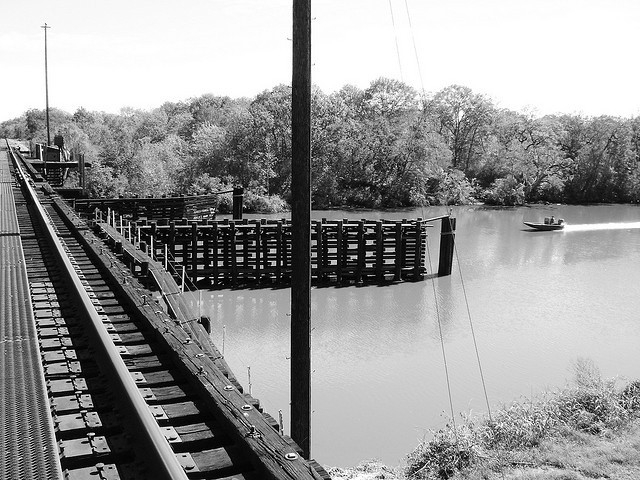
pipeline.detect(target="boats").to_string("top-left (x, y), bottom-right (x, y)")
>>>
top-left (523, 222), bottom-right (565, 230)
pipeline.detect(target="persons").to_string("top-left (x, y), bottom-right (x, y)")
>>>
top-left (549, 216), bottom-right (555, 225)
top-left (54, 131), bottom-right (70, 162)
